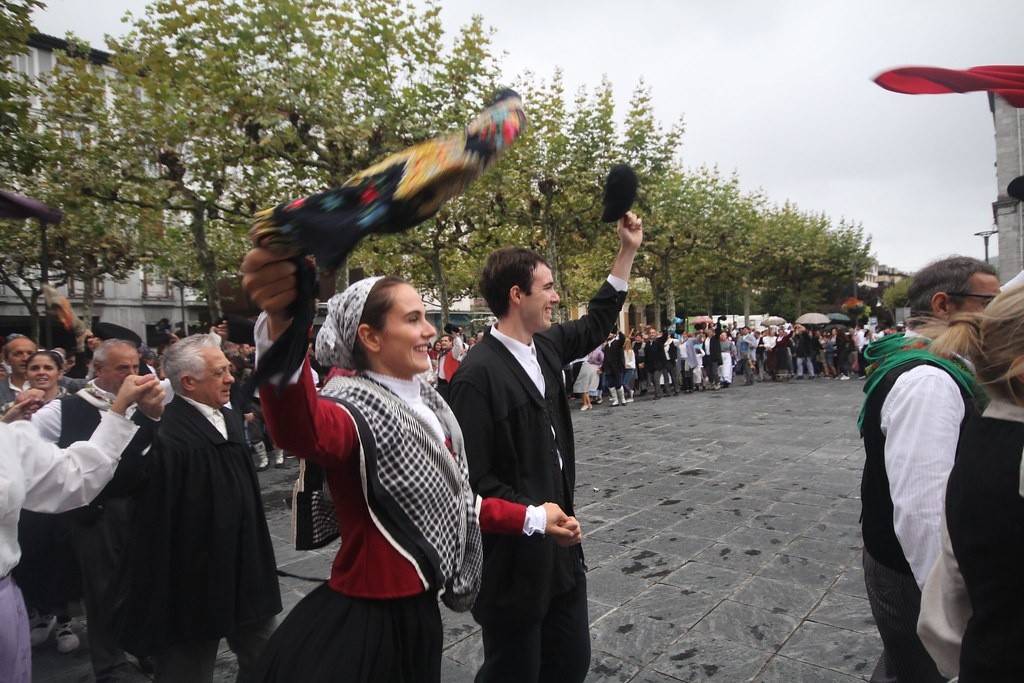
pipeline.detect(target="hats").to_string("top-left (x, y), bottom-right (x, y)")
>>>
top-left (445, 323), bottom-right (459, 334)
top-left (663, 319), bottom-right (671, 326)
top-left (695, 324), bottom-right (701, 330)
top-left (90, 320), bottom-right (141, 349)
top-left (601, 164), bottom-right (638, 223)
top-left (719, 316), bottom-right (726, 320)
top-left (609, 326), bottom-right (616, 334)
top-left (676, 328), bottom-right (684, 334)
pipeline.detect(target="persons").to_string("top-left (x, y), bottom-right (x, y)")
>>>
top-left (858, 255), bottom-right (1024, 683)
top-left (912, 285), bottom-right (1024, 683)
top-left (422, 315), bottom-right (905, 413)
top-left (235, 244), bottom-right (575, 683)
top-left (0, 284), bottom-right (362, 683)
top-left (451, 210), bottom-right (643, 683)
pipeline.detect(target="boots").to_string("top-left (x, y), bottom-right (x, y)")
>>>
top-left (272, 445), bottom-right (285, 468)
top-left (617, 386), bottom-right (626, 406)
top-left (253, 441), bottom-right (270, 472)
top-left (608, 387), bottom-right (618, 406)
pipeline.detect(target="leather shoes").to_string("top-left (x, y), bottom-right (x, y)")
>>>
top-left (139, 656), bottom-right (153, 673)
top-left (97, 662), bottom-right (153, 683)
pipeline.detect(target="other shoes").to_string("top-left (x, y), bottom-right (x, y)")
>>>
top-left (286, 453), bottom-right (296, 458)
top-left (30, 616), bottom-right (56, 647)
top-left (580, 395), bottom-right (603, 411)
top-left (630, 390), bottom-right (634, 397)
top-left (642, 370), bottom-right (868, 399)
top-left (56, 622), bottom-right (79, 653)
top-left (626, 398), bottom-right (634, 402)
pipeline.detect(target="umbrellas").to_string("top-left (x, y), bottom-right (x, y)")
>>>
top-left (826, 313), bottom-right (851, 321)
top-left (795, 312), bottom-right (831, 324)
